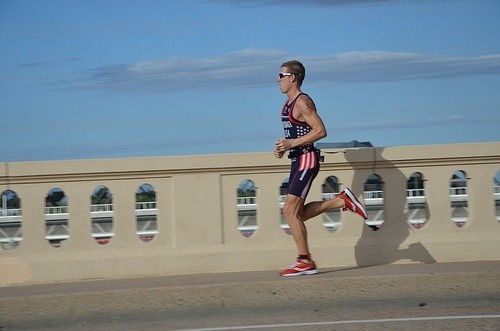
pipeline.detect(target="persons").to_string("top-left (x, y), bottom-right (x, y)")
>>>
top-left (272, 60), bottom-right (368, 276)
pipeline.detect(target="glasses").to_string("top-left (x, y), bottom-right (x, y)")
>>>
top-left (278, 72), bottom-right (302, 79)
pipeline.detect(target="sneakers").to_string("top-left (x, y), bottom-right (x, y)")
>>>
top-left (279, 256), bottom-right (318, 277)
top-left (338, 187), bottom-right (368, 219)
